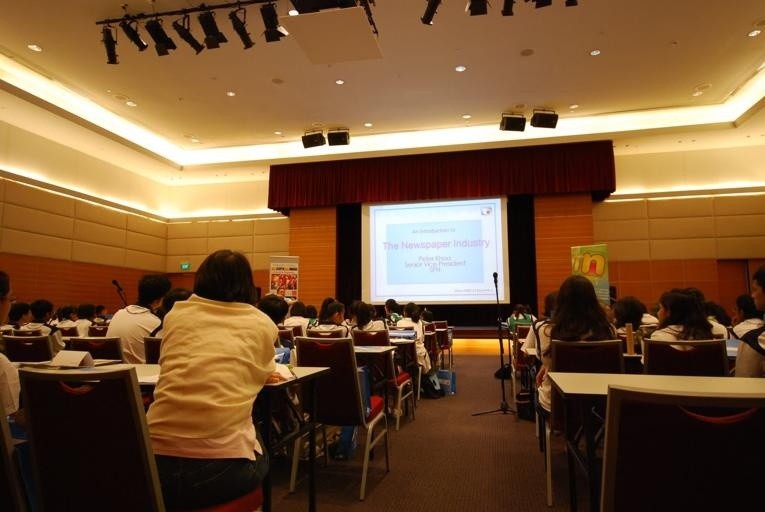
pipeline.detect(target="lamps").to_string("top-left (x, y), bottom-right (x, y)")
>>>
top-left (120, 20), bottom-right (148, 51)
top-left (500, 112), bottom-right (526, 133)
top-left (101, 23), bottom-right (120, 63)
top-left (530, 109), bottom-right (560, 129)
top-left (260, 3), bottom-right (289, 42)
top-left (535, 0), bottom-right (552, 9)
top-left (565, 0), bottom-right (578, 7)
top-left (420, 0), bottom-right (442, 27)
top-left (328, 127), bottom-right (350, 146)
top-left (198, 12), bottom-right (228, 49)
top-left (465, 0), bottom-right (492, 18)
top-left (501, 0), bottom-right (515, 17)
top-left (302, 130), bottom-right (326, 149)
top-left (143, 19), bottom-right (177, 57)
top-left (171, 16), bottom-right (205, 55)
top-left (229, 8), bottom-right (255, 52)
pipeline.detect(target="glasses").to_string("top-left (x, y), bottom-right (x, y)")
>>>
top-left (8, 295), bottom-right (16, 303)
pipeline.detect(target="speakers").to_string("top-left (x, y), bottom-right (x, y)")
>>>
top-left (500, 116), bottom-right (526, 132)
top-left (530, 113), bottom-right (558, 129)
top-left (327, 132), bottom-right (350, 146)
top-left (302, 132), bottom-right (326, 148)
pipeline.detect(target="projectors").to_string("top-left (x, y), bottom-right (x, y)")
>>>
top-left (277, 1), bottom-right (385, 66)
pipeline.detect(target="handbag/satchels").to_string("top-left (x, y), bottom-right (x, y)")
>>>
top-left (357, 366), bottom-right (370, 416)
top-left (437, 370), bottom-right (456, 395)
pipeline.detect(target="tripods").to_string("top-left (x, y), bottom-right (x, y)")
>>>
top-left (471, 284), bottom-right (518, 416)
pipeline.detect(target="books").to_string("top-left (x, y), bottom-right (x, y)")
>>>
top-left (19, 350), bottom-right (122, 370)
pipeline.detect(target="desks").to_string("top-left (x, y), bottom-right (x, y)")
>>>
top-left (298, 342), bottom-right (396, 461)
top-left (548, 372), bottom-right (765, 512)
top-left (0, 362), bottom-right (329, 512)
top-left (525, 347), bottom-right (538, 421)
top-left (517, 338), bottom-right (526, 344)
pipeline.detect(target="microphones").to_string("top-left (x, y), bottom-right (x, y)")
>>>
top-left (493, 272), bottom-right (497, 284)
top-left (112, 280), bottom-right (124, 291)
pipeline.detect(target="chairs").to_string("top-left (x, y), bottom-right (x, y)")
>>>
top-left (389, 326), bottom-right (424, 401)
top-left (354, 329), bottom-right (414, 430)
top-left (278, 329), bottom-right (295, 349)
top-left (59, 327), bottom-right (79, 335)
top-left (20, 366), bottom-right (269, 512)
top-left (425, 324), bottom-right (442, 371)
top-left (88, 326), bottom-right (108, 336)
top-left (552, 340), bottom-right (624, 375)
top-left (308, 329), bottom-right (346, 337)
top-left (290, 336), bottom-right (391, 502)
top-left (13, 329), bottom-right (41, 337)
top-left (69, 337), bottom-right (123, 361)
top-left (433, 320), bottom-right (453, 369)
top-left (0, 397), bottom-right (25, 512)
top-left (510, 322), bottom-right (532, 412)
top-left (4, 337), bottom-right (52, 361)
top-left (645, 337), bottom-right (729, 377)
top-left (145, 338), bottom-right (164, 363)
top-left (278, 324), bottom-right (303, 336)
top-left (599, 384), bottom-right (765, 511)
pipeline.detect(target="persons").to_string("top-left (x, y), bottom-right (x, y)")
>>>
top-left (8, 300), bottom-right (112, 354)
top-left (730, 292), bottom-right (763, 340)
top-left (611, 294), bottom-right (643, 368)
top-left (163, 289), bottom-right (191, 314)
top-left (147, 250), bottom-right (290, 506)
top-left (0, 271), bottom-right (36, 512)
top-left (734, 266), bottom-right (765, 376)
top-left (705, 299), bottom-right (734, 342)
top-left (508, 301), bottom-right (535, 336)
top-left (256, 289), bottom-right (433, 374)
top-left (643, 288), bottom-right (719, 352)
top-left (105, 274), bottom-right (171, 365)
top-left (517, 291), bottom-right (554, 363)
top-left (538, 274), bottom-right (618, 477)
